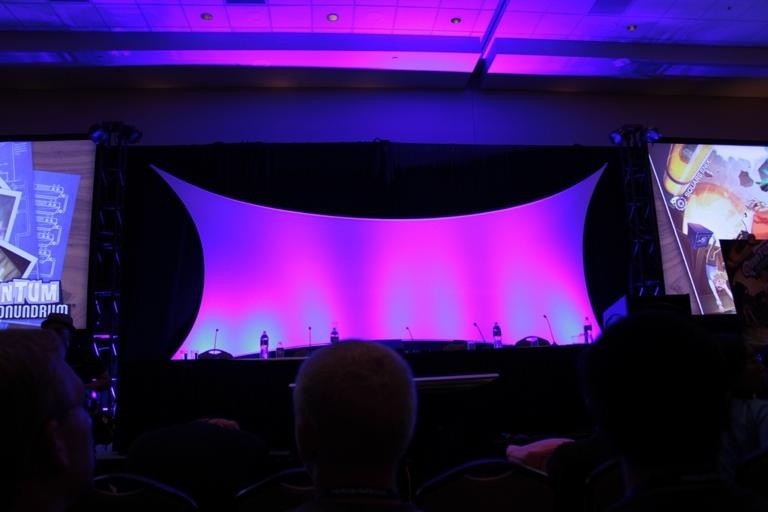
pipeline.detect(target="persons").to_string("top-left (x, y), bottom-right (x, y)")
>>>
top-left (0, 308), bottom-right (768, 511)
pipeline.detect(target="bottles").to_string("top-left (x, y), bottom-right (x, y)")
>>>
top-left (491, 321), bottom-right (503, 349)
top-left (275, 341), bottom-right (285, 359)
top-left (259, 329), bottom-right (269, 360)
top-left (329, 327), bottom-right (339, 344)
top-left (582, 316), bottom-right (593, 344)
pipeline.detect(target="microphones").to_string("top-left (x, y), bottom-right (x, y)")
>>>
top-left (213, 329), bottom-right (218, 348)
top-left (543, 314), bottom-right (557, 345)
top-left (406, 326), bottom-right (414, 340)
top-left (308, 326), bottom-right (312, 346)
top-left (473, 322), bottom-right (485, 342)
